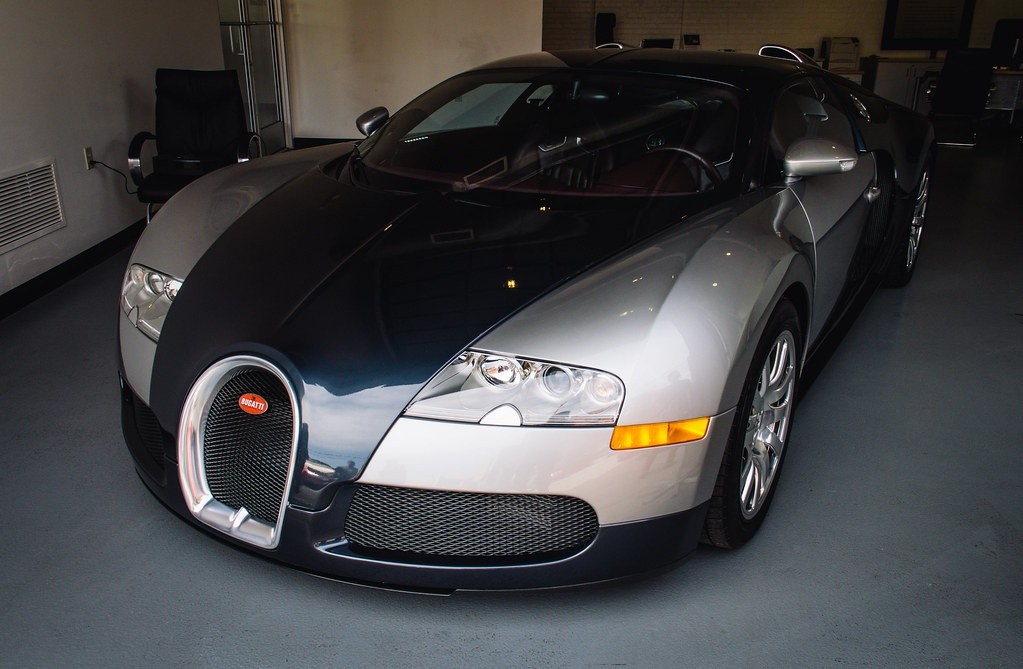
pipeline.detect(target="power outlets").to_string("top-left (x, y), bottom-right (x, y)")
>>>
top-left (84, 147), bottom-right (95, 169)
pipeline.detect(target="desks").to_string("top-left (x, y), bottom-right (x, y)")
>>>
top-left (871, 55), bottom-right (1023, 127)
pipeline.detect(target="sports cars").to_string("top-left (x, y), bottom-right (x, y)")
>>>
top-left (114, 40), bottom-right (936, 598)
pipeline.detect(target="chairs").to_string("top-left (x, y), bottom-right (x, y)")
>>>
top-left (498, 87), bottom-right (772, 192)
top-left (127, 68), bottom-right (267, 227)
top-left (923, 47), bottom-right (996, 148)
top-left (682, 33), bottom-right (702, 52)
top-left (969, 19), bottom-right (1023, 71)
top-left (593, 12), bottom-right (624, 51)
